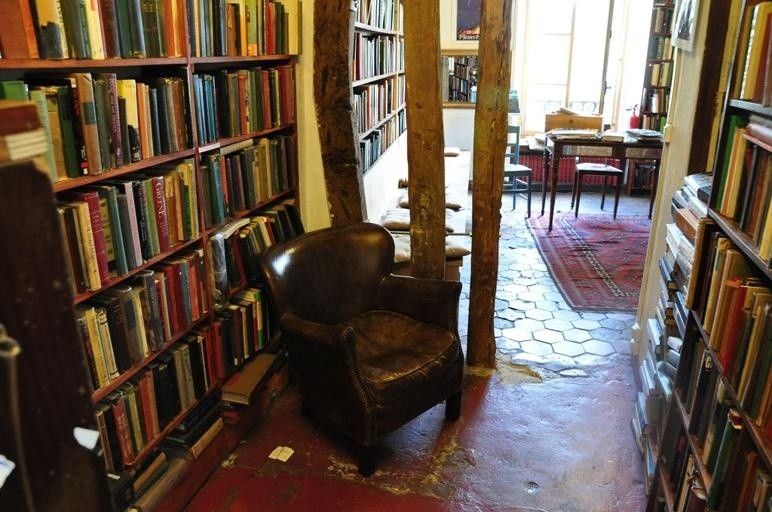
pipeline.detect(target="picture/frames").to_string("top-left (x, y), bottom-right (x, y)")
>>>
top-left (452, 0), bottom-right (481, 44)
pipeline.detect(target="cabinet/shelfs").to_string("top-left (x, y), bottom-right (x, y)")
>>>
top-left (0, 53), bottom-right (300, 512)
top-left (631, 1), bottom-right (771, 512)
top-left (351, 21), bottom-right (408, 180)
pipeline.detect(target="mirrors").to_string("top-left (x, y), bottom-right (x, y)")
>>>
top-left (438, 50), bottom-right (480, 108)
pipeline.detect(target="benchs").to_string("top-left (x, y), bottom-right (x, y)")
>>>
top-left (377, 146), bottom-right (473, 282)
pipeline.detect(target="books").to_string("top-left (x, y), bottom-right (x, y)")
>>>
top-left (0, 0), bottom-right (304, 512)
top-left (448, 56), bottom-right (476, 101)
top-left (352, 1), bottom-right (407, 171)
top-left (630, 0), bottom-right (772, 512)
top-left (509, 0), bottom-right (677, 192)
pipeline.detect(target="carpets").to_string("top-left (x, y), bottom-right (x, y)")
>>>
top-left (526, 211), bottom-right (651, 312)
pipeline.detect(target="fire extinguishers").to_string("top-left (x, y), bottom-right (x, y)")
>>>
top-left (626, 104), bottom-right (640, 129)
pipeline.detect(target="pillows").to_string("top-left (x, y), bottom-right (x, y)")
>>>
top-left (399, 191), bottom-right (462, 209)
top-left (383, 208), bottom-right (455, 232)
top-left (399, 177), bottom-right (450, 188)
top-left (393, 237), bottom-right (471, 265)
top-left (443, 146), bottom-right (460, 157)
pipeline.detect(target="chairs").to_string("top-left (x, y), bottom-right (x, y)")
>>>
top-left (570, 156), bottom-right (624, 220)
top-left (257, 223), bottom-right (464, 479)
top-left (503, 125), bottom-right (533, 218)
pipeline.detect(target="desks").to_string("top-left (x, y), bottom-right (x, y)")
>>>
top-left (541, 132), bottom-right (664, 232)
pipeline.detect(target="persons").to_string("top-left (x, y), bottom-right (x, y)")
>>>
top-left (469, 57), bottom-right (479, 85)
